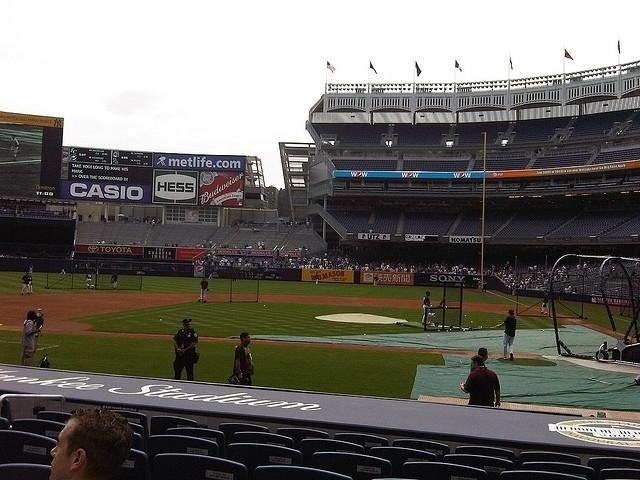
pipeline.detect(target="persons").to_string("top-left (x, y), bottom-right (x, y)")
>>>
top-left (540, 290), bottom-right (550, 315)
top-left (60, 266), bottom-right (66, 275)
top-left (48, 407), bottom-right (132, 480)
top-left (20, 310), bottom-right (38, 366)
top-left (20, 260), bottom-right (34, 296)
top-left (469, 347), bottom-right (488, 374)
top-left (231, 332), bottom-right (255, 387)
top-left (493, 309), bottom-right (517, 361)
top-left (82, 232), bottom-right (640, 305)
top-left (634, 374), bottom-right (640, 385)
top-left (420, 289), bottom-right (434, 325)
top-left (596, 340), bottom-right (609, 360)
top-left (173, 319), bottom-right (199, 380)
top-left (34, 308), bottom-right (43, 337)
top-left (8, 134), bottom-right (21, 161)
top-left (459, 354), bottom-right (500, 409)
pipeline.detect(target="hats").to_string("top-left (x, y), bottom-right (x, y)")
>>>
top-left (183, 318), bottom-right (192, 323)
top-left (509, 309), bottom-right (514, 313)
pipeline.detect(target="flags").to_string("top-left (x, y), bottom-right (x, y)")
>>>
top-left (370, 61), bottom-right (378, 75)
top-left (618, 41), bottom-right (622, 53)
top-left (415, 61), bottom-right (422, 76)
top-left (455, 59), bottom-right (463, 71)
top-left (509, 58), bottom-right (514, 69)
top-left (326, 60), bottom-right (335, 73)
top-left (564, 48), bottom-right (573, 60)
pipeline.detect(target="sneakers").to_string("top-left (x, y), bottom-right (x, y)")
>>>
top-left (510, 352), bottom-right (514, 361)
top-left (504, 357), bottom-right (508, 359)
